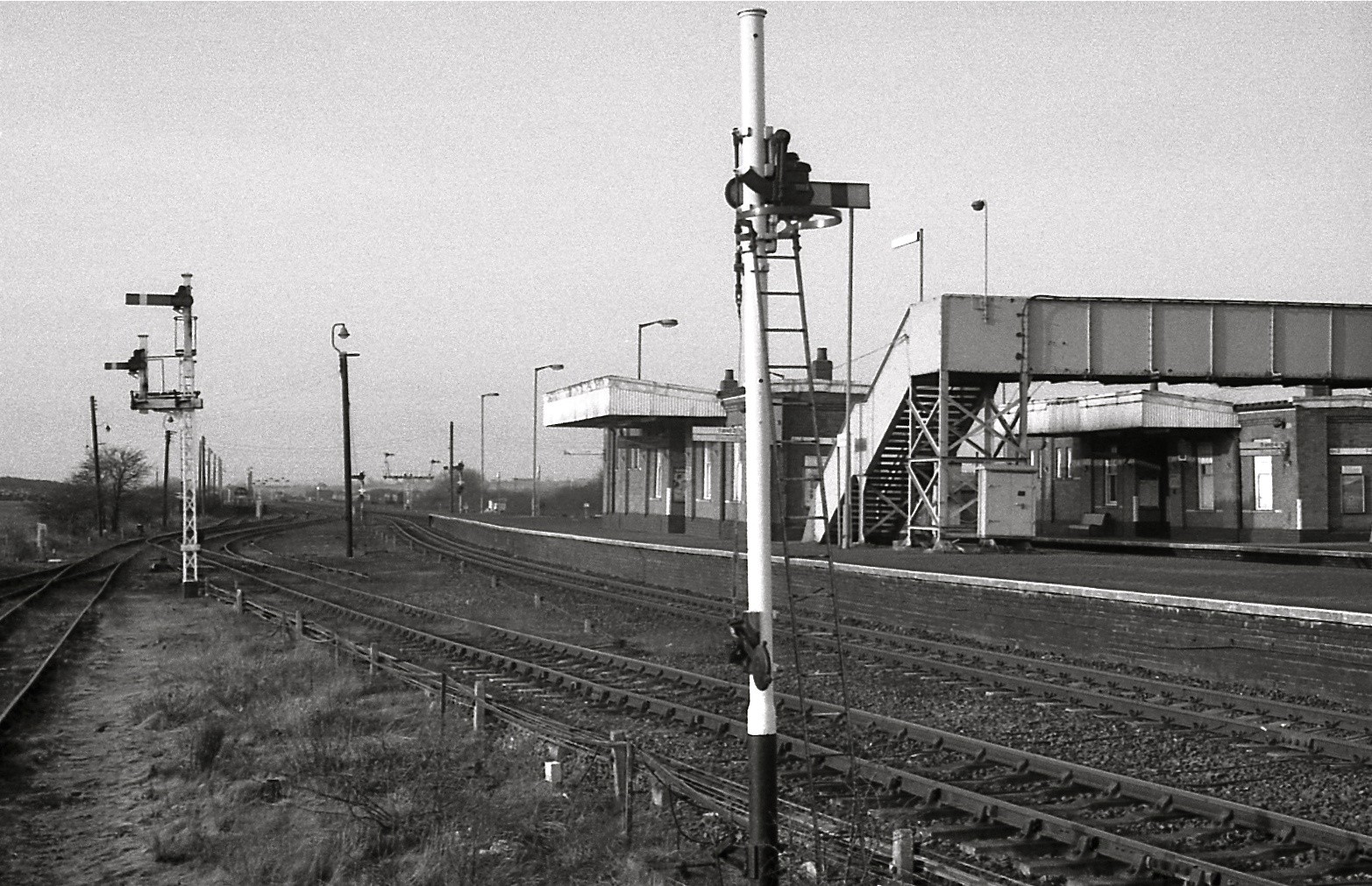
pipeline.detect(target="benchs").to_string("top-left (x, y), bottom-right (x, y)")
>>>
top-left (1066, 512), bottom-right (1112, 539)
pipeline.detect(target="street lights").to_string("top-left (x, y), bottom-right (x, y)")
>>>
top-left (91, 421), bottom-right (111, 538)
top-left (531, 363), bottom-right (565, 519)
top-left (330, 321), bottom-right (360, 557)
top-left (637, 318), bottom-right (678, 380)
top-left (971, 199), bottom-right (989, 322)
top-left (161, 413), bottom-right (178, 528)
top-left (480, 392), bottom-right (500, 514)
